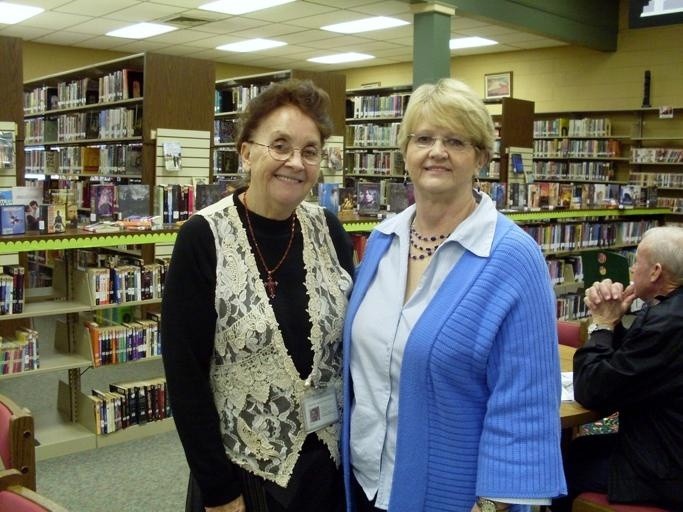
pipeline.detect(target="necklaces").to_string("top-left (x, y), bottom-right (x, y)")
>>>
top-left (409, 221), bottom-right (452, 261)
top-left (243, 189), bottom-right (296, 301)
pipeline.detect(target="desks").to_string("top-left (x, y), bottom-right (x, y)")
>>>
top-left (556, 342), bottom-right (625, 428)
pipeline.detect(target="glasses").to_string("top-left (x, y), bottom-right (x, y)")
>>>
top-left (407, 131), bottom-right (475, 152)
top-left (249, 139), bottom-right (329, 167)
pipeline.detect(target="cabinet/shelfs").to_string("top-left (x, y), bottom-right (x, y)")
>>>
top-left (22, 53), bottom-right (214, 213)
top-left (490, 108), bottom-right (683, 208)
top-left (1, 211), bottom-right (672, 463)
top-left (215, 71), bottom-right (346, 197)
top-left (345, 85), bottom-right (411, 193)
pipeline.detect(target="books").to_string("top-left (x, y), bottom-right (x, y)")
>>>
top-left (214, 82), bottom-right (277, 174)
top-left (345, 93), bottom-right (414, 200)
top-left (0, 265), bottom-right (24, 315)
top-left (521, 220), bottom-right (681, 324)
top-left (474, 115), bottom-right (683, 215)
top-left (351, 234), bottom-right (369, 267)
top-left (85, 312), bottom-right (161, 363)
top-left (1, 328), bottom-right (41, 375)
top-left (86, 377), bottom-right (174, 433)
top-left (151, 182), bottom-right (241, 230)
top-left (84, 260), bottom-right (171, 305)
top-left (1, 67), bottom-right (151, 233)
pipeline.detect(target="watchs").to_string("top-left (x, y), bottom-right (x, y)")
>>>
top-left (473, 495), bottom-right (510, 512)
top-left (586, 324), bottom-right (613, 335)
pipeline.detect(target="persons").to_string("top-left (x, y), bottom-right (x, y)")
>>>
top-left (540, 225), bottom-right (682, 510)
top-left (340, 75), bottom-right (567, 512)
top-left (158, 75), bottom-right (354, 511)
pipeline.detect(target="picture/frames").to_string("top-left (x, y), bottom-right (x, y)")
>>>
top-left (484, 71), bottom-right (514, 104)
